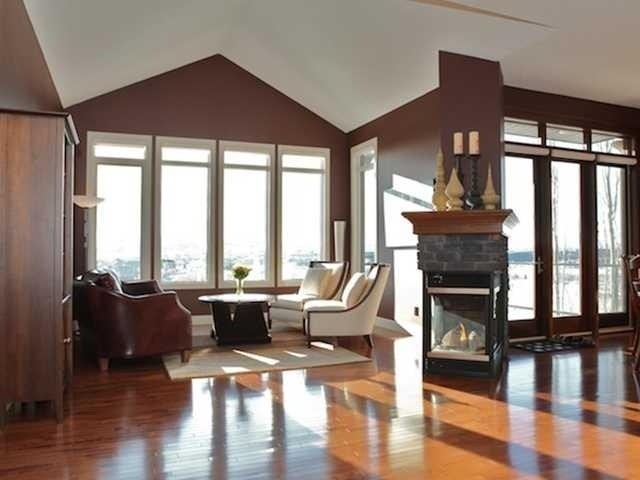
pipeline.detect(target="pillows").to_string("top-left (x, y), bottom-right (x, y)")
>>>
top-left (341, 272), bottom-right (367, 308)
top-left (297, 267), bottom-right (333, 297)
top-left (119, 280), bottom-right (160, 293)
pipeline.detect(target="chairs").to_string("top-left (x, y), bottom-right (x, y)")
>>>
top-left (268, 260), bottom-right (349, 335)
top-left (303, 263), bottom-right (392, 348)
top-left (622, 254), bottom-right (640, 372)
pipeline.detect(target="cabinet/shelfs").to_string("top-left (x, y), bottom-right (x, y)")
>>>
top-left (0, 106), bottom-right (81, 423)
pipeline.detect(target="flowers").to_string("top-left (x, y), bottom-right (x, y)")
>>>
top-left (234, 266), bottom-right (253, 292)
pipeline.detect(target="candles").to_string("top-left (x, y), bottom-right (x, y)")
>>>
top-left (453, 132), bottom-right (463, 154)
top-left (469, 131), bottom-right (480, 155)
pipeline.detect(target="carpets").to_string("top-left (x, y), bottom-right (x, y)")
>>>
top-left (162, 332), bottom-right (373, 380)
top-left (509, 338), bottom-right (578, 354)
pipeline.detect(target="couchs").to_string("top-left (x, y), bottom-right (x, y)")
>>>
top-left (72, 267), bottom-right (192, 372)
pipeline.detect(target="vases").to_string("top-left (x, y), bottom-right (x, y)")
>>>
top-left (236, 278), bottom-right (244, 296)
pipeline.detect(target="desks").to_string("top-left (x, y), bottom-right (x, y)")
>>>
top-left (197, 293), bottom-right (272, 347)
